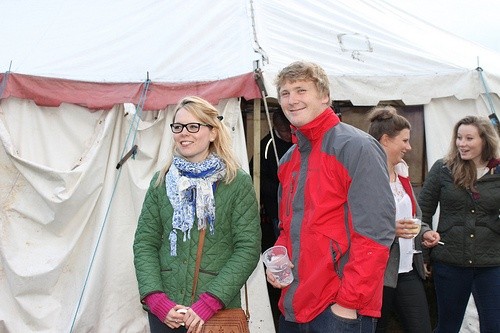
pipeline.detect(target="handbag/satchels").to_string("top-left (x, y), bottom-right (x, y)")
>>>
top-left (192, 308), bottom-right (250, 333)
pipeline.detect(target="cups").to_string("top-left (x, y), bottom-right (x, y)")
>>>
top-left (261, 246), bottom-right (293, 286)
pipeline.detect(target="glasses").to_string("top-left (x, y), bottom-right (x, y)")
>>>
top-left (170, 122), bottom-right (209, 133)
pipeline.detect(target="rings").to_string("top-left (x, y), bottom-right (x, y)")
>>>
top-left (199, 322), bottom-right (204, 325)
top-left (175, 319), bottom-right (178, 323)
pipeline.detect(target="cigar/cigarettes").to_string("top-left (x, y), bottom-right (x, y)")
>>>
top-left (435, 239), bottom-right (446, 245)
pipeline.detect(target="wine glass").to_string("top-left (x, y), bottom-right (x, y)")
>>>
top-left (404, 215), bottom-right (422, 253)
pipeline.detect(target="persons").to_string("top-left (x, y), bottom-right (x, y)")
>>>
top-left (414, 113), bottom-right (500, 333)
top-left (241, 103), bottom-right (305, 333)
top-left (132, 95), bottom-right (263, 333)
top-left (363, 104), bottom-right (442, 333)
top-left (264, 59), bottom-right (396, 332)
